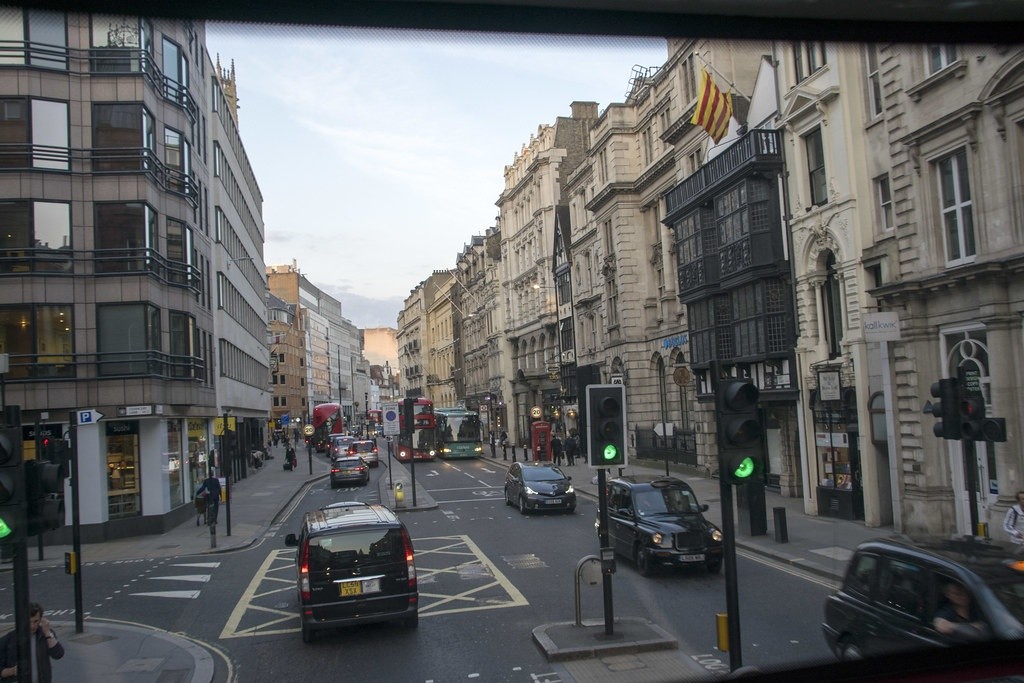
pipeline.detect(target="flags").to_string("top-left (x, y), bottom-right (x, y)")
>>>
top-left (690, 67), bottom-right (733, 145)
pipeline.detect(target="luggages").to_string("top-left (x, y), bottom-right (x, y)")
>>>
top-left (283, 459), bottom-right (291, 470)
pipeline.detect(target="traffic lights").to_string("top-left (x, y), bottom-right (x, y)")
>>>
top-left (586, 383), bottom-right (629, 470)
top-left (24, 458), bottom-right (64, 537)
top-left (719, 376), bottom-right (762, 484)
top-left (930, 379), bottom-right (962, 440)
top-left (0, 425), bottom-right (24, 546)
top-left (40, 437), bottom-right (56, 459)
top-left (959, 393), bottom-right (1007, 442)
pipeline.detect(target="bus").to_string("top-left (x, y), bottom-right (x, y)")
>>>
top-left (314, 403), bottom-right (343, 453)
top-left (368, 409), bottom-right (383, 425)
top-left (433, 408), bottom-right (484, 460)
top-left (389, 397), bottom-right (436, 462)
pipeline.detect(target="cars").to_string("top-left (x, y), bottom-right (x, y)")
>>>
top-left (343, 441), bottom-right (378, 468)
top-left (325, 433), bottom-right (377, 463)
top-left (504, 462), bottom-right (578, 515)
top-left (821, 531), bottom-right (1024, 664)
top-left (330, 456), bottom-right (371, 488)
top-left (595, 474), bottom-right (723, 576)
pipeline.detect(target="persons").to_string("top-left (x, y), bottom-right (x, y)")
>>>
top-left (572, 432), bottom-right (581, 458)
top-left (550, 436), bottom-right (563, 466)
top-left (1002, 491), bottom-right (1024, 545)
top-left (564, 434), bottom-right (577, 466)
top-left (933, 580), bottom-right (987, 637)
top-left (293, 428), bottom-right (299, 444)
top-left (500, 430), bottom-right (508, 445)
top-left (272, 433), bottom-right (290, 448)
top-left (196, 471), bottom-right (222, 526)
top-left (286, 444), bottom-right (295, 471)
top-left (0, 602), bottom-right (65, 683)
top-left (488, 431), bottom-right (493, 445)
top-left (193, 476), bottom-right (210, 526)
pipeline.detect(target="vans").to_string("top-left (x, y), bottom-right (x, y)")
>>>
top-left (285, 500), bottom-right (419, 644)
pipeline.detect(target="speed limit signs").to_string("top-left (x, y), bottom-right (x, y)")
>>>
top-left (530, 406), bottom-right (543, 419)
top-left (302, 424), bottom-right (315, 437)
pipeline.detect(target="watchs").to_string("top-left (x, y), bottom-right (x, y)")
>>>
top-left (46, 633), bottom-right (55, 640)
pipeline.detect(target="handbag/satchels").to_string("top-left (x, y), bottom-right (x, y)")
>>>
top-left (293, 457), bottom-right (297, 468)
top-left (560, 451), bottom-right (564, 459)
